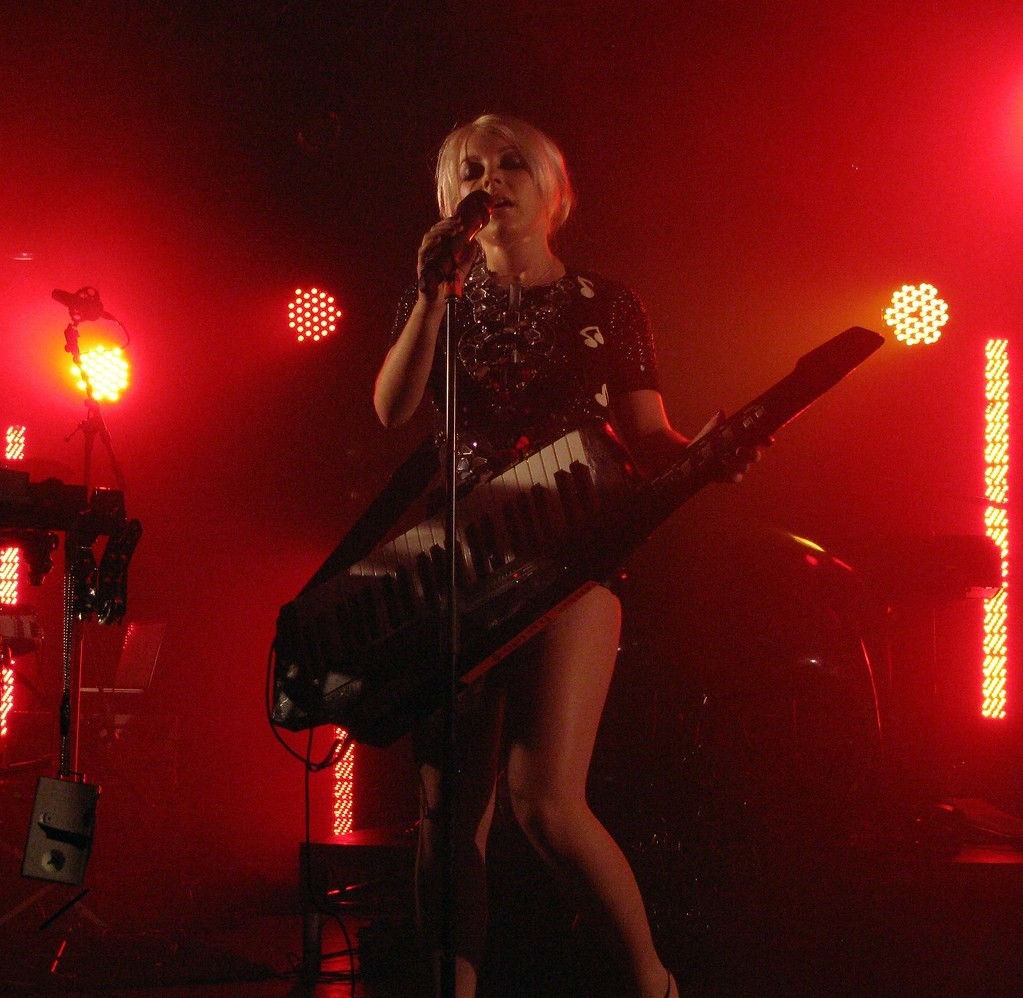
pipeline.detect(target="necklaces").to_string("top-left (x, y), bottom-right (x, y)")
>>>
top-left (484, 258), bottom-right (557, 289)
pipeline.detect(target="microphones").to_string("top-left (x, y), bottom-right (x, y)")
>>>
top-left (50, 288), bottom-right (114, 320)
top-left (422, 190), bottom-right (494, 276)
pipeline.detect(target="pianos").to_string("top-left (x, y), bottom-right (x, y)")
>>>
top-left (269, 424), bottom-right (631, 735)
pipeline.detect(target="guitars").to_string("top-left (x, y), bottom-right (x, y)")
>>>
top-left (337, 318), bottom-right (891, 750)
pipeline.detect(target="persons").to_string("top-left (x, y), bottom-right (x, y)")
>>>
top-left (373, 115), bottom-right (776, 998)
